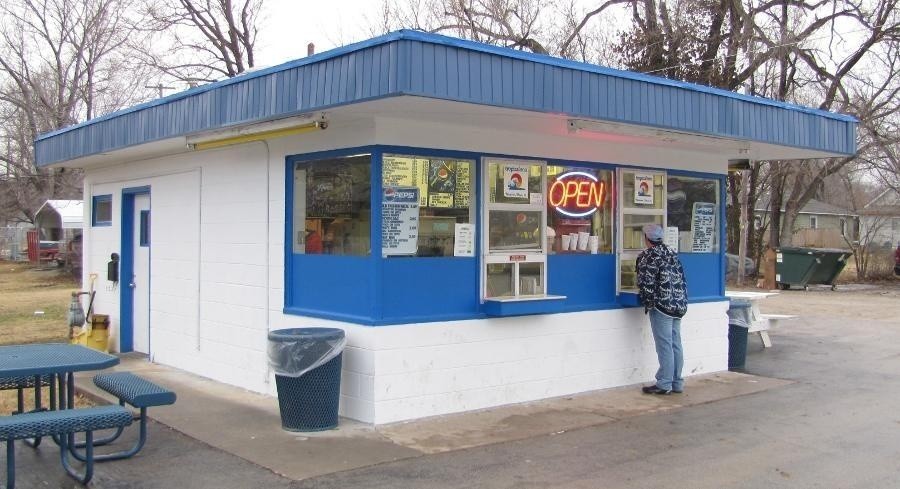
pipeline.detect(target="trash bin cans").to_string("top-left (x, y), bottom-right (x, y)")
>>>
top-left (268, 327), bottom-right (345, 431)
top-left (728, 302), bottom-right (751, 369)
top-left (771, 247), bottom-right (853, 291)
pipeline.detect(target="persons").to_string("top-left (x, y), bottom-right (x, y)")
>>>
top-left (634, 223), bottom-right (688, 396)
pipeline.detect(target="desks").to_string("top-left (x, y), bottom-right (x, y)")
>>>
top-left (724, 289), bottom-right (780, 348)
top-left (0, 343), bottom-right (120, 484)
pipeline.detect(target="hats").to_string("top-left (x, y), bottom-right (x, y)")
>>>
top-left (305, 220), bottom-right (315, 232)
top-left (641, 224), bottom-right (665, 242)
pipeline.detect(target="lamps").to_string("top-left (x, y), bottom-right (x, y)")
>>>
top-left (186, 113), bottom-right (326, 153)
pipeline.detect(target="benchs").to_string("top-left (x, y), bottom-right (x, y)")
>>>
top-left (756, 314), bottom-right (799, 348)
top-left (0, 370), bottom-right (177, 489)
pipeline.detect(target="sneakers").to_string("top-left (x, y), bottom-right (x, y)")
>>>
top-left (642, 385), bottom-right (682, 395)
